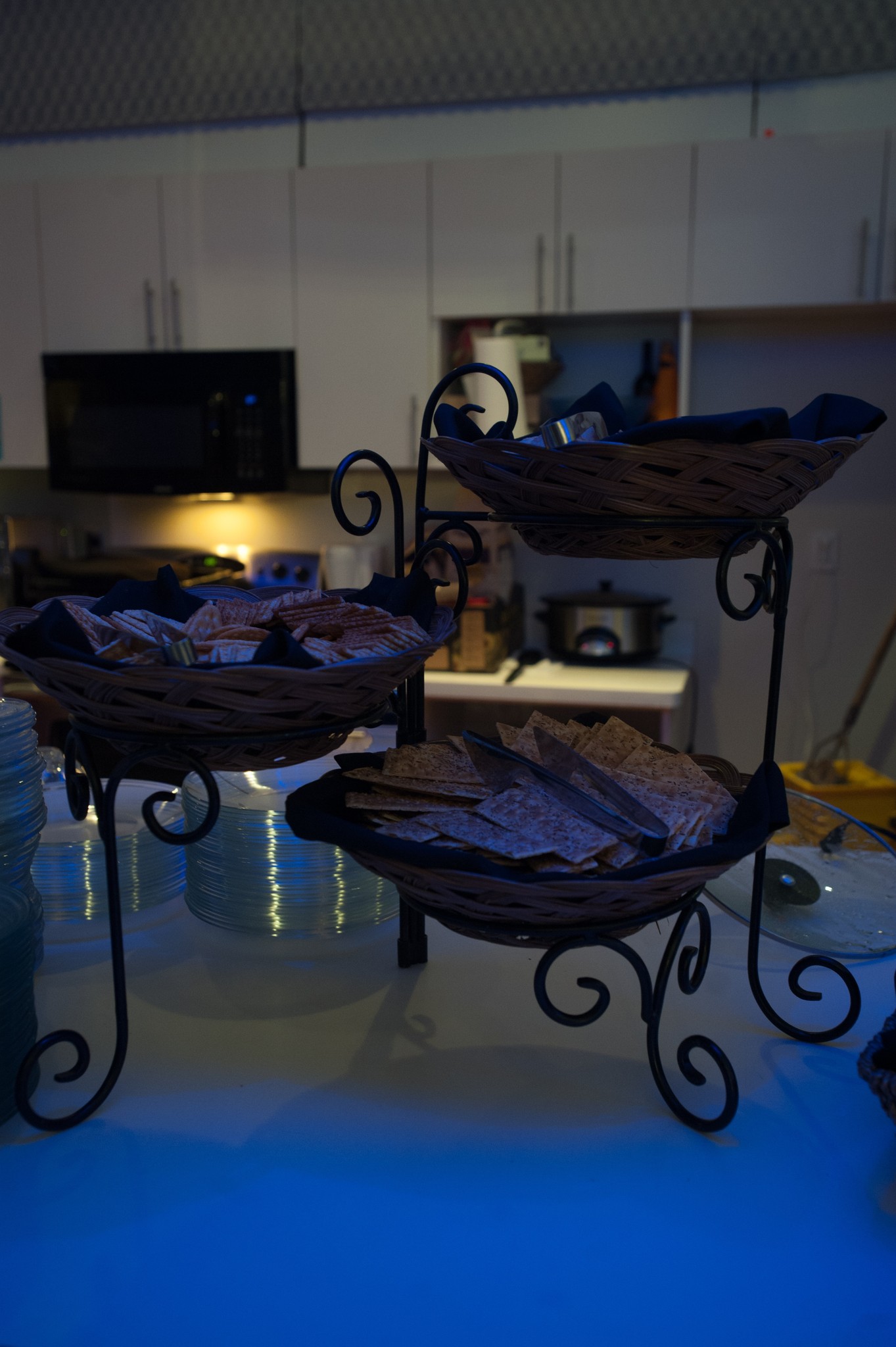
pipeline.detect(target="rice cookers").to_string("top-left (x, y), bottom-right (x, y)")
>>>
top-left (538, 574), bottom-right (680, 666)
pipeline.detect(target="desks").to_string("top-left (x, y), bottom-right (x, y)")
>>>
top-left (425, 653), bottom-right (692, 753)
top-left (4, 742), bottom-right (893, 1347)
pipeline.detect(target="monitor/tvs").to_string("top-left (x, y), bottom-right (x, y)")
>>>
top-left (37, 345), bottom-right (300, 499)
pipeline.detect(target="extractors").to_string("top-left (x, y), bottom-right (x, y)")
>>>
top-left (38, 333), bottom-right (328, 510)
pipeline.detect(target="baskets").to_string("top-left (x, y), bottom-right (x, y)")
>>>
top-left (421, 432), bottom-right (876, 561)
top-left (340, 741), bottom-right (776, 951)
top-left (1, 580), bottom-right (446, 775)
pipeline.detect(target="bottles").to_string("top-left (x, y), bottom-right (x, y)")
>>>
top-left (630, 331), bottom-right (675, 428)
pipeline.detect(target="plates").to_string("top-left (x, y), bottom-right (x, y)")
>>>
top-left (0, 682), bottom-right (409, 1135)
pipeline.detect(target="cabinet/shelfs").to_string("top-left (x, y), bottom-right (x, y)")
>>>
top-left (704, 496), bottom-right (895, 796)
top-left (0, 115), bottom-right (894, 484)
